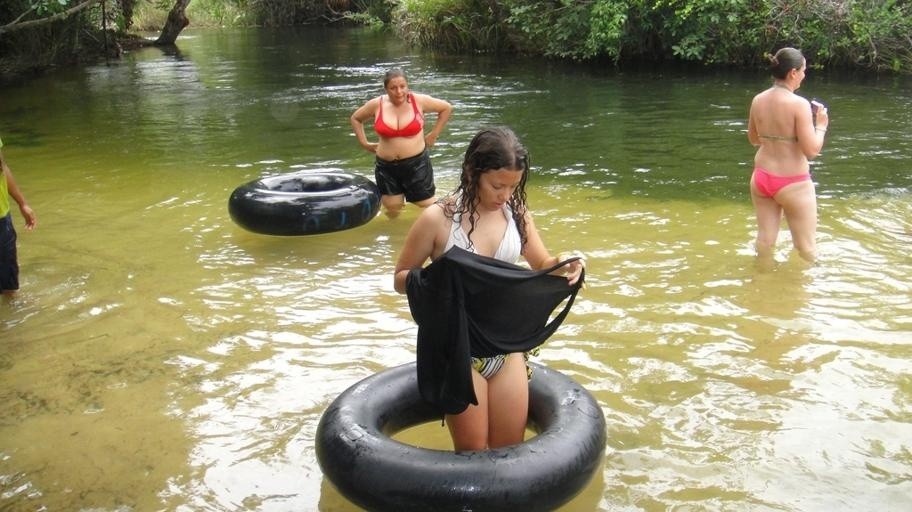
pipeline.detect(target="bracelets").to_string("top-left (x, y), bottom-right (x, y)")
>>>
top-left (813, 126), bottom-right (827, 132)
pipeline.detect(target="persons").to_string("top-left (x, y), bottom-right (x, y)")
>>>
top-left (1, 139), bottom-right (38, 300)
top-left (351, 68), bottom-right (452, 212)
top-left (748, 46), bottom-right (829, 260)
top-left (395, 126), bottom-right (588, 453)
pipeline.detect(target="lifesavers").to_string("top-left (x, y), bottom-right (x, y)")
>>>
top-left (314, 357), bottom-right (605, 511)
top-left (227, 170), bottom-right (379, 235)
